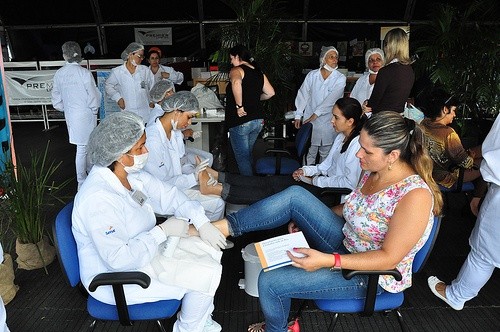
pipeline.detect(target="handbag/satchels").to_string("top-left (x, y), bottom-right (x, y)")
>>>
top-left (404, 102), bottom-right (424, 123)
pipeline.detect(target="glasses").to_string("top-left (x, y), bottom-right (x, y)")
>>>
top-left (137, 54), bottom-right (145, 59)
top-left (151, 57), bottom-right (159, 60)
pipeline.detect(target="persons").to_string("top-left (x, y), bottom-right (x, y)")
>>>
top-left (428, 109), bottom-right (500, 312)
top-left (291, 28), bottom-right (485, 216)
top-left (51, 42), bottom-right (275, 224)
top-left (71, 110), bottom-right (225, 332)
top-left (186, 112), bottom-right (442, 332)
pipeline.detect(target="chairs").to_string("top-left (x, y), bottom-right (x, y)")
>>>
top-left (51, 123), bottom-right (475, 332)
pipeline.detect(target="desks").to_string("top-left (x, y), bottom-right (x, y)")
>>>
top-left (185, 110), bottom-right (226, 153)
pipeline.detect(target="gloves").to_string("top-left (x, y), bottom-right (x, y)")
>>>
top-left (193, 157), bottom-right (210, 181)
top-left (206, 172), bottom-right (222, 187)
top-left (160, 215), bottom-right (191, 237)
top-left (199, 222), bottom-right (228, 252)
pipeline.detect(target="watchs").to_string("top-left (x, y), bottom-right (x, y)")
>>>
top-left (330, 254), bottom-right (342, 273)
top-left (235, 104), bottom-right (243, 109)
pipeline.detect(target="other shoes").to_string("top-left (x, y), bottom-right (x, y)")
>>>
top-left (172, 310), bottom-right (222, 332)
top-left (428, 276), bottom-right (463, 311)
top-left (247, 318), bottom-right (300, 332)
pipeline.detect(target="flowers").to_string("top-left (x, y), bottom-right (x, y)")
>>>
top-left (0, 140), bottom-right (75, 274)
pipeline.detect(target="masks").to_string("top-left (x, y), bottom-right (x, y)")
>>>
top-left (369, 69), bottom-right (378, 75)
top-left (170, 111), bottom-right (180, 130)
top-left (131, 60), bottom-right (140, 67)
top-left (121, 153), bottom-right (149, 174)
top-left (324, 64), bottom-right (338, 72)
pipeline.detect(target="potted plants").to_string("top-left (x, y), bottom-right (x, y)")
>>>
top-left (409, 0), bottom-right (500, 150)
